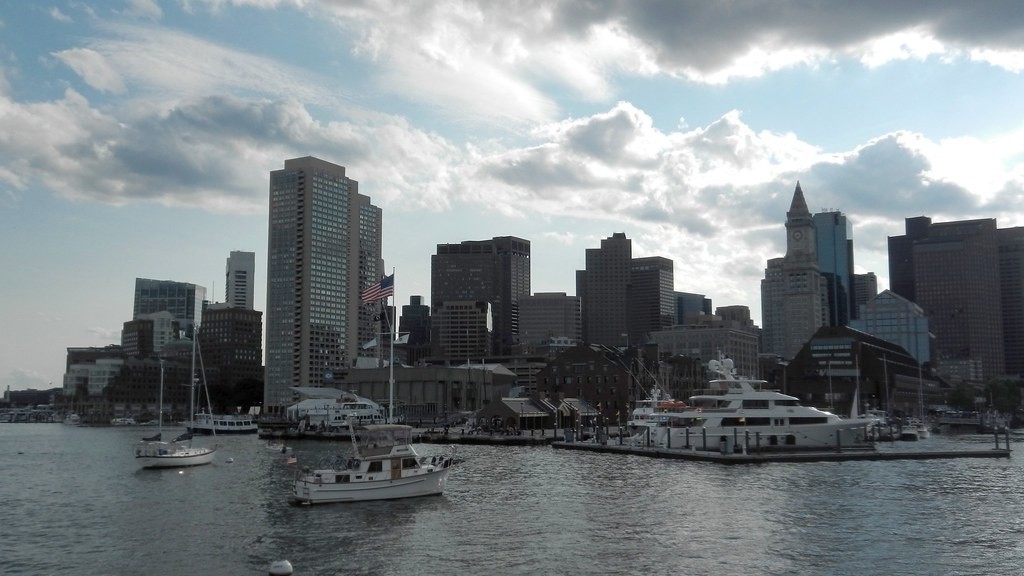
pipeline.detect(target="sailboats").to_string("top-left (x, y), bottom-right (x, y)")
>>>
top-left (185, 323), bottom-right (259, 433)
top-left (132, 367), bottom-right (219, 468)
top-left (290, 424), bottom-right (456, 511)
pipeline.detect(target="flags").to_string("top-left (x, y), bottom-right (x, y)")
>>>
top-left (361, 274), bottom-right (393, 305)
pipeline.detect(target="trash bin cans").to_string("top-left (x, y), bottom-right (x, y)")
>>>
top-left (565, 430), bottom-right (574, 442)
top-left (719, 435), bottom-right (727, 453)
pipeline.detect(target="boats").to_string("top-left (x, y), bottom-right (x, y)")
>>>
top-left (635, 350), bottom-right (881, 448)
